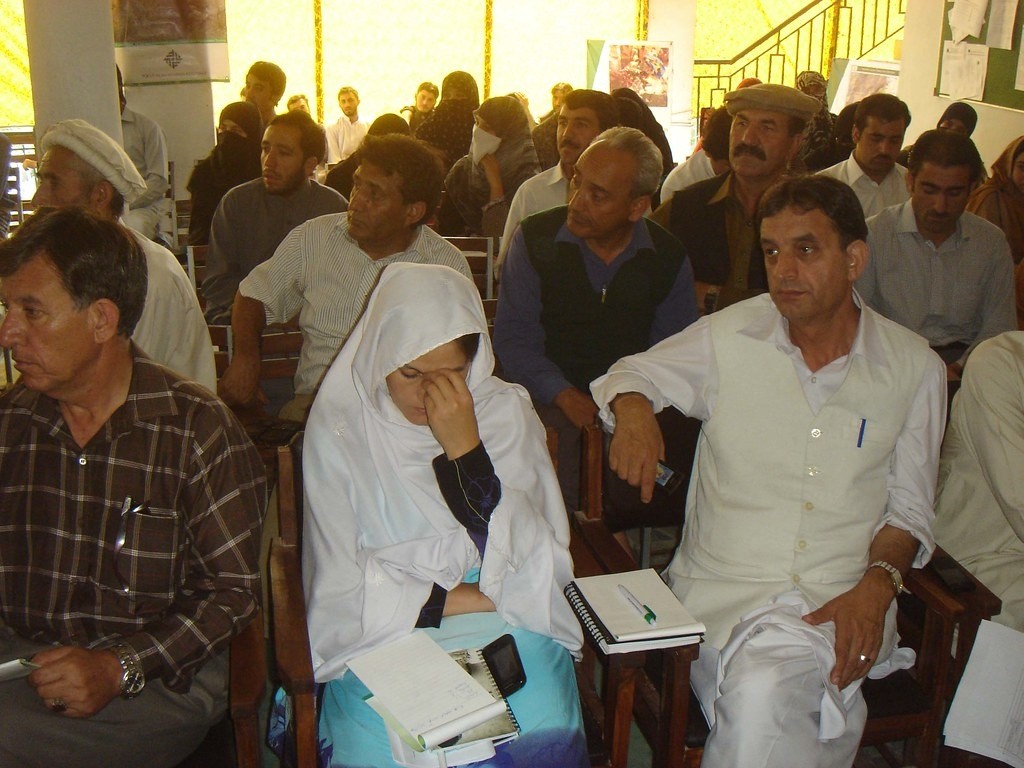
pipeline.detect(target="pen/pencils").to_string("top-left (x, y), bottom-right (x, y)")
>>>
top-left (617, 583), bottom-right (658, 624)
top-left (857, 418), bottom-right (866, 447)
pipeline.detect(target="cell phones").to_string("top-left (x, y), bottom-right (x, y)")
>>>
top-left (928, 556), bottom-right (977, 594)
top-left (482, 634), bottom-right (527, 697)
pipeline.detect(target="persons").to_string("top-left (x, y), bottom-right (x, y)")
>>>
top-left (216, 134), bottom-right (471, 477)
top-left (302, 261), bottom-right (587, 768)
top-left (32, 118), bottom-right (218, 398)
top-left (0, 206), bottom-right (267, 768)
top-left (23, 63), bottom-right (168, 241)
top-left (191, 64), bottom-right (1024, 400)
top-left (0, 130), bottom-right (18, 241)
top-left (933, 326), bottom-right (1024, 637)
top-left (496, 128), bottom-right (703, 531)
top-left (588, 177), bottom-right (949, 768)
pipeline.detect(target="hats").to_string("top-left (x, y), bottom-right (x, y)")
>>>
top-left (552, 82), bottom-right (573, 93)
top-left (723, 84), bottom-right (822, 121)
top-left (39, 118), bottom-right (147, 203)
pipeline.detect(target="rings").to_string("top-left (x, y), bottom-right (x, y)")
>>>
top-left (51, 697), bottom-right (68, 713)
top-left (860, 654), bottom-right (871, 662)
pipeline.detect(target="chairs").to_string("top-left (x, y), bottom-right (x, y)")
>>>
top-left (0, 159), bottom-right (1009, 768)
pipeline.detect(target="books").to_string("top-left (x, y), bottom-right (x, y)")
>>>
top-left (564, 568), bottom-right (706, 655)
top-left (345, 626), bottom-right (523, 768)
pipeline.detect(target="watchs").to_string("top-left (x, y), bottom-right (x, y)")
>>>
top-left (864, 560), bottom-right (904, 596)
top-left (92, 636), bottom-right (146, 700)
